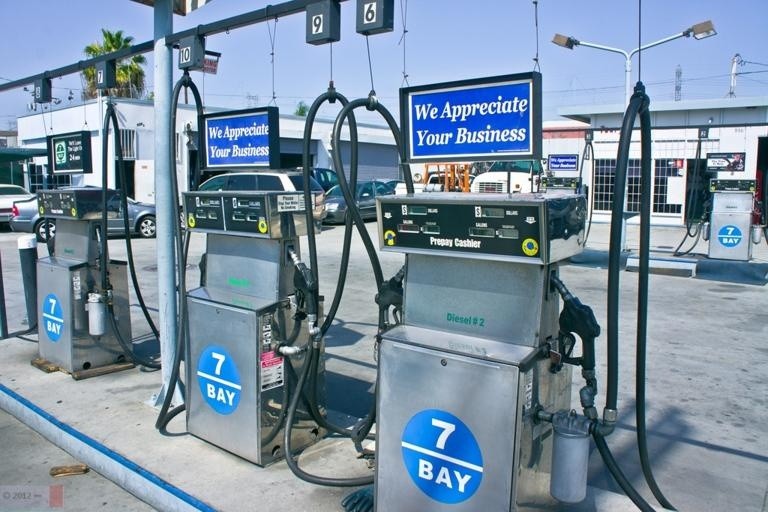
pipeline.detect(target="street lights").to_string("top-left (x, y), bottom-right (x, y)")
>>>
top-left (552, 19), bottom-right (718, 254)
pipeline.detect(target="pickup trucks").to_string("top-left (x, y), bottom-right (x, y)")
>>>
top-left (395, 173), bottom-right (476, 194)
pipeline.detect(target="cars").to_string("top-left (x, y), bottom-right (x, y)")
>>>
top-left (471, 158), bottom-right (546, 194)
top-left (1, 184), bottom-right (156, 243)
top-left (180, 168), bottom-right (395, 230)
